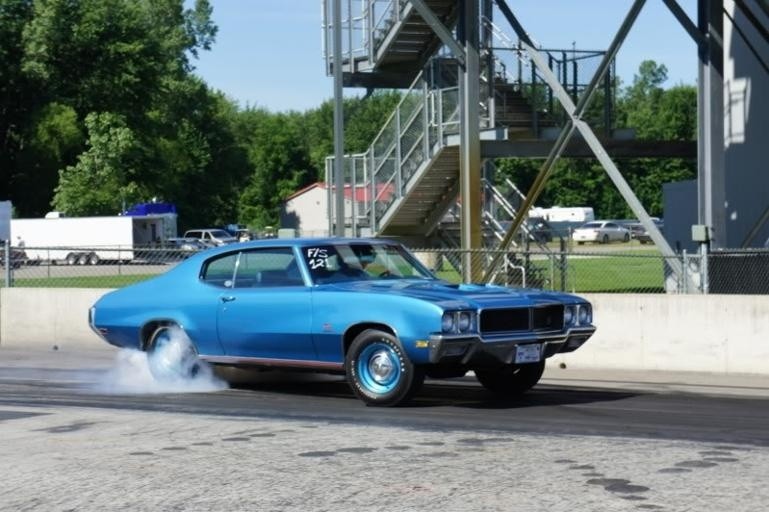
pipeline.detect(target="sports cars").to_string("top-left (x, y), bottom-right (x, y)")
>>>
top-left (89, 237), bottom-right (598, 405)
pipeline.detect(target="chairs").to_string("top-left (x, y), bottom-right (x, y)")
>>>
top-left (256, 267), bottom-right (287, 287)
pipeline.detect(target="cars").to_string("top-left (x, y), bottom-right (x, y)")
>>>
top-left (498, 220), bottom-right (553, 244)
top-left (0, 244), bottom-right (28, 270)
top-left (632, 217), bottom-right (664, 244)
top-left (572, 220), bottom-right (631, 246)
top-left (166, 229), bottom-right (240, 258)
